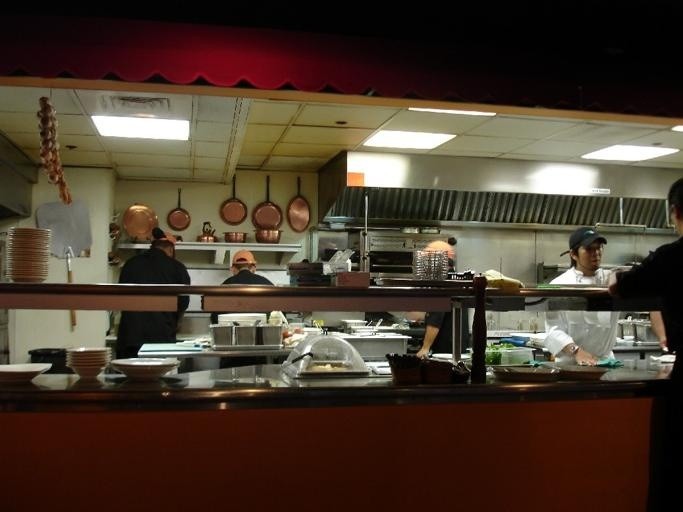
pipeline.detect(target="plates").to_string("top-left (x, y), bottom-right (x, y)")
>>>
top-left (411, 250), bottom-right (449, 281)
top-left (4, 226), bottom-right (51, 283)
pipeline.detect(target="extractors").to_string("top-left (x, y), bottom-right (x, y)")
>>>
top-left (316, 150), bottom-right (683, 236)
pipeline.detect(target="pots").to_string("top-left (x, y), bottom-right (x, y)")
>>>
top-left (252, 227), bottom-right (283, 243)
top-left (223, 231), bottom-right (247, 243)
top-left (218, 173), bottom-right (247, 225)
top-left (166, 187), bottom-right (190, 230)
top-left (286, 177), bottom-right (311, 232)
top-left (122, 203), bottom-right (157, 243)
top-left (251, 176), bottom-right (282, 230)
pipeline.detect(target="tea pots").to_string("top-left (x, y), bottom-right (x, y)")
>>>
top-left (196, 221), bottom-right (218, 242)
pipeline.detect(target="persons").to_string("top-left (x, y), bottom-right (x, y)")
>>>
top-left (115, 228), bottom-right (190, 374)
top-left (545, 226), bottom-right (628, 368)
top-left (647, 310), bottom-right (678, 357)
top-left (211, 250), bottom-right (276, 369)
top-left (607, 177), bottom-right (682, 512)
top-left (416, 237), bottom-right (471, 358)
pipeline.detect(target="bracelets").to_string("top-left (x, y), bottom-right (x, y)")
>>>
top-left (566, 346), bottom-right (581, 356)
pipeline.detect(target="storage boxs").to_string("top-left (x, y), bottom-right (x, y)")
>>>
top-left (501, 347), bottom-right (537, 367)
top-left (465, 346), bottom-right (501, 365)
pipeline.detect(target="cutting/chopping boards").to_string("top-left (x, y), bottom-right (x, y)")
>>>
top-left (139, 343), bottom-right (202, 352)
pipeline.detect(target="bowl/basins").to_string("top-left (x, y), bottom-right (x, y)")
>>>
top-left (109, 357), bottom-right (180, 381)
top-left (303, 328), bottom-right (320, 337)
top-left (559, 365), bottom-right (608, 382)
top-left (0, 363), bottom-right (52, 383)
top-left (64, 347), bottom-right (112, 380)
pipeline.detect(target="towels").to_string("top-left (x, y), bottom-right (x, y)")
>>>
top-left (591, 356), bottom-right (623, 368)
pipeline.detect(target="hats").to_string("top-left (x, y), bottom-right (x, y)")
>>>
top-left (234, 250), bottom-right (256, 264)
top-left (569, 227), bottom-right (607, 250)
top-left (150, 232), bottom-right (175, 244)
top-left (424, 240), bottom-right (455, 260)
